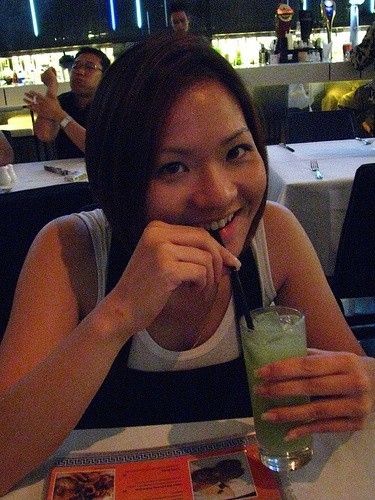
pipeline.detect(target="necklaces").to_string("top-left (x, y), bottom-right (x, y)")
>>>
top-left (193, 283), bottom-right (223, 349)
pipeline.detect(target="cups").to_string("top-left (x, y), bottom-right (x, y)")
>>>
top-left (240, 307), bottom-right (314, 472)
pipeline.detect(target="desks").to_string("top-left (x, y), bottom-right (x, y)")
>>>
top-left (266, 137), bottom-right (375, 277)
top-left (0, 158), bottom-right (89, 194)
top-left (0, 417), bottom-right (375, 500)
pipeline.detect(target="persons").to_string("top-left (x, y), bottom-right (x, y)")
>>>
top-left (0, 36), bottom-right (375, 496)
top-left (23, 47), bottom-right (110, 160)
top-left (0, 131), bottom-right (14, 166)
top-left (170, 5), bottom-right (190, 34)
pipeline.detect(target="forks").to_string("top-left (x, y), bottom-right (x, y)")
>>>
top-left (355, 137), bottom-right (374, 145)
top-left (310, 161), bottom-right (323, 179)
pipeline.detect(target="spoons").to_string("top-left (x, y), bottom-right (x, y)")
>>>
top-left (280, 142), bottom-right (294, 152)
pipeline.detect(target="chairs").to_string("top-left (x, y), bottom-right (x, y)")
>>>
top-left (1, 182), bottom-right (94, 343)
top-left (281, 110), bottom-right (360, 144)
top-left (332, 163), bottom-right (375, 339)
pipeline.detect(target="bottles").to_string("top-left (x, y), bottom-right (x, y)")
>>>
top-left (259, 45), bottom-right (268, 64)
top-left (0, 165), bottom-right (16, 187)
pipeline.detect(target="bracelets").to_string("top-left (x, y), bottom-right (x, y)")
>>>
top-left (60, 114), bottom-right (72, 129)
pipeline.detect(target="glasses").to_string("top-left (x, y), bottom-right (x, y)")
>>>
top-left (71, 62), bottom-right (103, 73)
